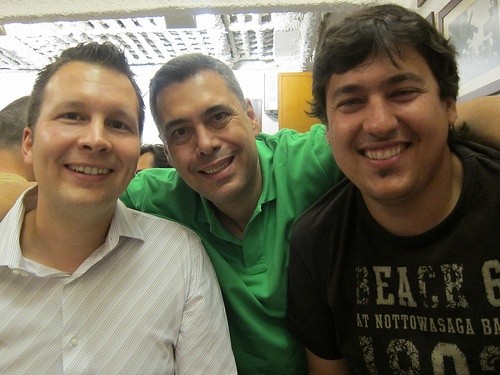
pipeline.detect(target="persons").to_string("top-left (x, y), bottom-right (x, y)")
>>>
top-left (0, 41), bottom-right (239, 375)
top-left (1, 94), bottom-right (42, 225)
top-left (133, 143), bottom-right (175, 179)
top-left (286, 2), bottom-right (500, 375)
top-left (1, 53), bottom-right (500, 375)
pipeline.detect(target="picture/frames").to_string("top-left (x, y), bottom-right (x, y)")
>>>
top-left (438, 0), bottom-right (500, 104)
